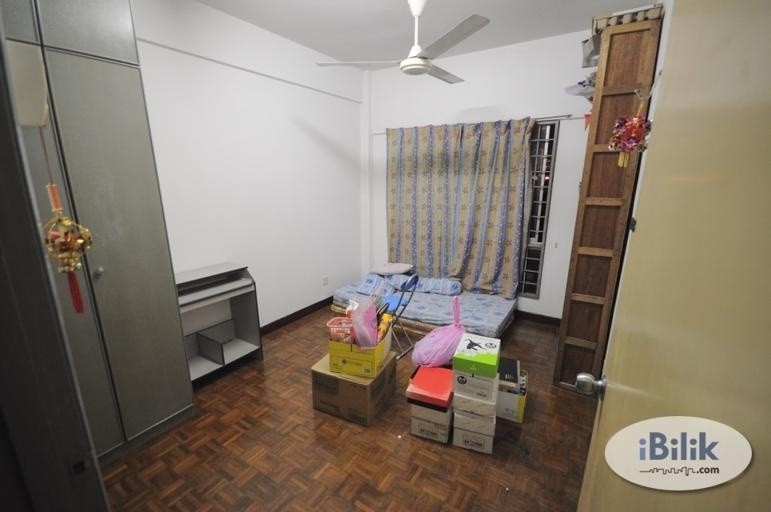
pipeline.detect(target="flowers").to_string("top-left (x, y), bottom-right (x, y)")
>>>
top-left (607, 117), bottom-right (653, 154)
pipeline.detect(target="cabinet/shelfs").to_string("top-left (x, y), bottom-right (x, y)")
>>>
top-left (551, 17), bottom-right (661, 397)
top-left (1, 0), bottom-right (196, 467)
top-left (175, 263), bottom-right (264, 388)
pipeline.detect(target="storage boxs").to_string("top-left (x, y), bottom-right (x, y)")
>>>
top-left (405, 332), bottom-right (529, 454)
top-left (311, 314), bottom-right (397, 427)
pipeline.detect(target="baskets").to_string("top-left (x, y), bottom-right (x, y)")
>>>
top-left (326, 317), bottom-right (354, 342)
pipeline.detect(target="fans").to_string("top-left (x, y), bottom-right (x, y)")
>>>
top-left (314, 0), bottom-right (490, 84)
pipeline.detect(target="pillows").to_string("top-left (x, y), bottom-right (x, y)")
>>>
top-left (358, 263), bottom-right (414, 298)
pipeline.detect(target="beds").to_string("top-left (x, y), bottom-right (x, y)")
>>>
top-left (331, 271), bottom-right (517, 340)
